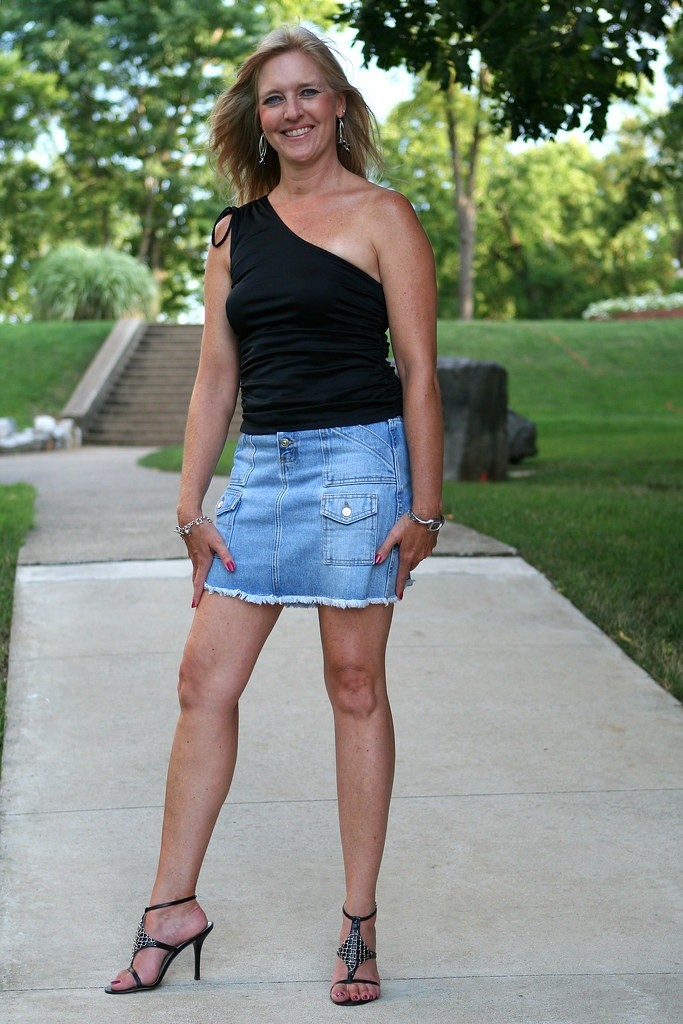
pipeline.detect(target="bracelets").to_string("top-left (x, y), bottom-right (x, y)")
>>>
top-left (172, 514), bottom-right (210, 538)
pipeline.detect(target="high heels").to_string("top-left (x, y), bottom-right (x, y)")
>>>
top-left (103, 893), bottom-right (214, 995)
top-left (329, 904), bottom-right (381, 1007)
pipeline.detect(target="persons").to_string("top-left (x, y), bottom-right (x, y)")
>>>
top-left (104, 19), bottom-right (445, 1007)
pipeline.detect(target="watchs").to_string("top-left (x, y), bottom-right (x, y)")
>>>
top-left (403, 509), bottom-right (446, 532)
